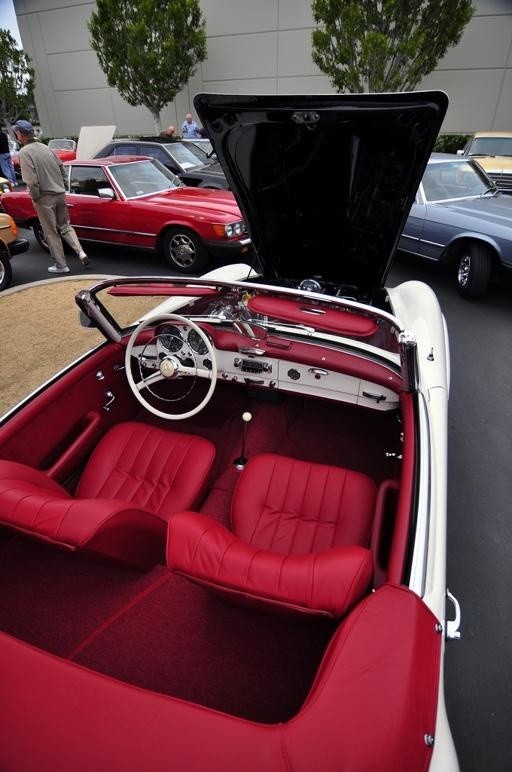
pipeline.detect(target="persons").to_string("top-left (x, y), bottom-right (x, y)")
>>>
top-left (179, 112), bottom-right (200, 138)
top-left (158, 126), bottom-right (175, 138)
top-left (11, 120), bottom-right (89, 274)
top-left (0, 129), bottom-right (18, 187)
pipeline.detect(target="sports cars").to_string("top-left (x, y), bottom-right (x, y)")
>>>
top-left (1, 91), bottom-right (460, 771)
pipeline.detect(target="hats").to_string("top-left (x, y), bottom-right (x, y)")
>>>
top-left (11, 120), bottom-right (34, 136)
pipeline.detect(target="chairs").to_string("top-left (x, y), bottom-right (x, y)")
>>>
top-left (0, 420), bottom-right (219, 575)
top-left (76, 174), bottom-right (100, 198)
top-left (113, 168), bottom-right (144, 200)
top-left (165, 452), bottom-right (379, 625)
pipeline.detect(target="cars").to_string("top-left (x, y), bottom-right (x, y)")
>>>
top-left (176, 139), bottom-right (218, 161)
top-left (1, 212), bottom-right (30, 292)
top-left (4, 133), bottom-right (39, 158)
top-left (394, 152), bottom-right (511, 299)
top-left (11, 139), bottom-right (77, 176)
top-left (1, 176), bottom-right (15, 196)
top-left (457, 132), bottom-right (511, 192)
top-left (1, 154), bottom-right (254, 274)
top-left (92, 139), bottom-right (232, 192)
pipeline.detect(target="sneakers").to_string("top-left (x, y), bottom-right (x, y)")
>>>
top-left (79, 253), bottom-right (89, 266)
top-left (48, 266), bottom-right (69, 273)
